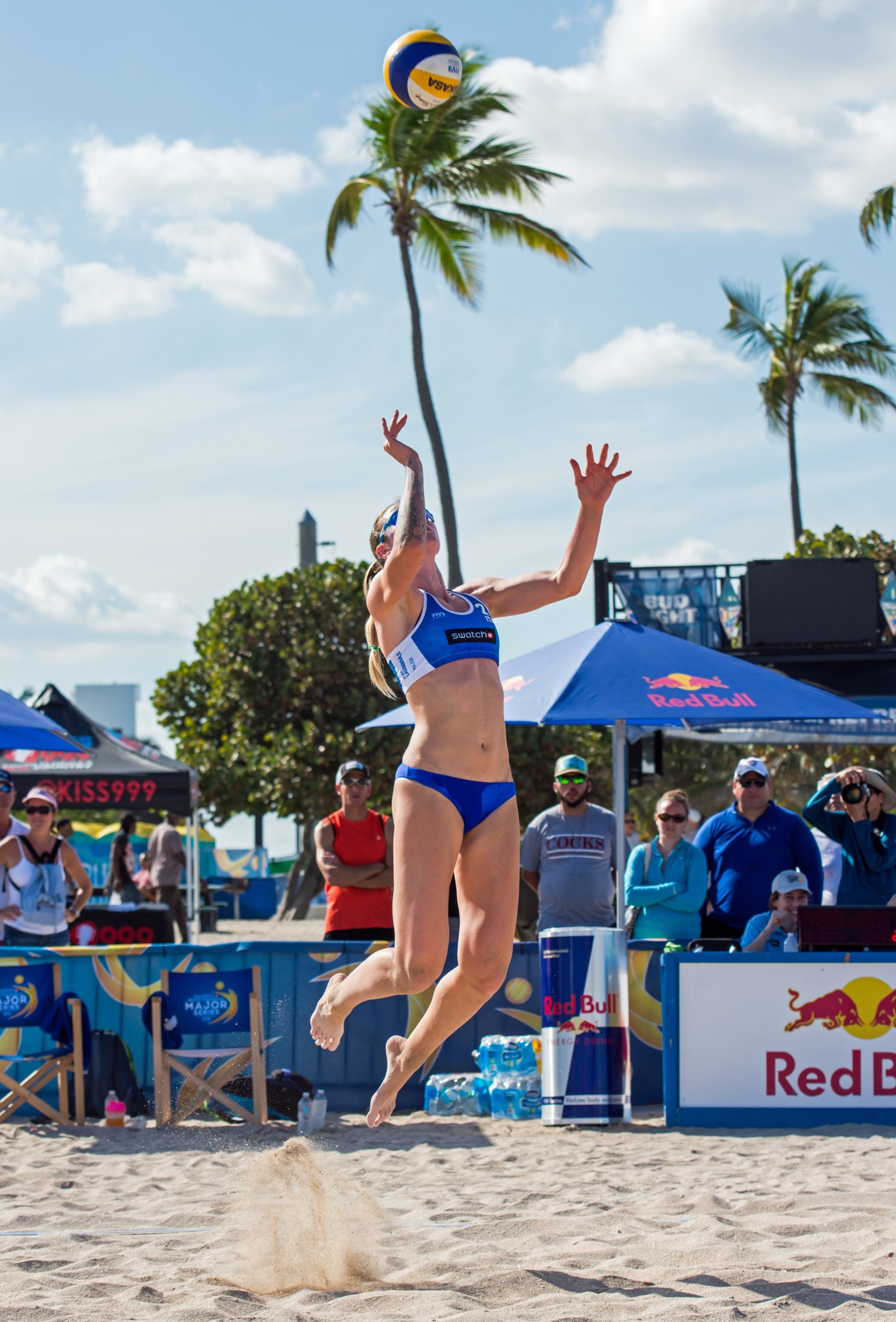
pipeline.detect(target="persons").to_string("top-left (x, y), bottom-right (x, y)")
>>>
top-left (623, 810), bottom-right (641, 851)
top-left (310, 411), bottom-right (632, 1131)
top-left (801, 765), bottom-right (896, 906)
top-left (314, 760), bottom-right (396, 940)
top-left (519, 754), bottom-right (632, 935)
top-left (105, 810), bottom-right (188, 943)
top-left (740, 869), bottom-right (813, 952)
top-left (0, 768), bottom-right (94, 946)
top-left (809, 772), bottom-right (851, 905)
top-left (681, 809), bottom-right (704, 846)
top-left (690, 757), bottom-right (824, 951)
top-left (618, 789), bottom-right (711, 954)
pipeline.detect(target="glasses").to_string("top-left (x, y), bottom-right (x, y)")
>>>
top-left (557, 774), bottom-right (586, 785)
top-left (658, 813), bottom-right (687, 823)
top-left (341, 778), bottom-right (369, 787)
top-left (736, 777), bottom-right (766, 788)
top-left (27, 805), bottom-right (50, 815)
top-left (379, 508), bottom-right (435, 563)
top-left (0, 785), bottom-right (12, 793)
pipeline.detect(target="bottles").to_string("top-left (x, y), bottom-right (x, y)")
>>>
top-left (297, 1092), bottom-right (313, 1135)
top-left (311, 1089), bottom-right (328, 1130)
top-left (425, 1034), bottom-right (540, 1120)
top-left (104, 1090), bottom-right (119, 1115)
top-left (126, 1115), bottom-right (146, 1129)
top-left (784, 932), bottom-right (798, 952)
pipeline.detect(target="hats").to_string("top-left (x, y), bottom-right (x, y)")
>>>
top-left (734, 757), bottom-right (770, 780)
top-left (554, 754), bottom-right (589, 777)
top-left (834, 765), bottom-right (896, 812)
top-left (0, 769), bottom-right (14, 785)
top-left (771, 870), bottom-right (813, 895)
top-left (335, 761), bottom-right (370, 785)
top-left (22, 788), bottom-right (58, 811)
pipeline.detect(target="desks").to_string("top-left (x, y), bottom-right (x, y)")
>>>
top-left (179, 883), bottom-right (241, 925)
top-left (66, 905), bottom-right (174, 947)
top-left (659, 951), bottom-right (896, 1129)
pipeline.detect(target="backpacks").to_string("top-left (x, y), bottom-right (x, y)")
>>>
top-left (196, 1069), bottom-right (314, 1125)
top-left (6, 834), bottom-right (66, 927)
top-left (68, 1029), bottom-right (151, 1116)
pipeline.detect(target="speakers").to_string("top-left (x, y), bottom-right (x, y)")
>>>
top-left (745, 557), bottom-right (880, 649)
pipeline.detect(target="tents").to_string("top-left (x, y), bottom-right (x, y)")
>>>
top-left (0, 684), bottom-right (200, 941)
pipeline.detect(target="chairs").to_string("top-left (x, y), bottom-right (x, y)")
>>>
top-left (0, 961), bottom-right (85, 1127)
top-left (150, 966), bottom-right (282, 1131)
top-left (687, 938), bottom-right (742, 952)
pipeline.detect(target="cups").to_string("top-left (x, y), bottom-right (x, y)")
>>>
top-left (106, 1101), bottom-right (126, 1127)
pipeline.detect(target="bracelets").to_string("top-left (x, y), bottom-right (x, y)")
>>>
top-left (69, 907), bottom-right (80, 917)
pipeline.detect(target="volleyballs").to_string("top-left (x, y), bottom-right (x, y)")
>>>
top-left (384, 29), bottom-right (463, 109)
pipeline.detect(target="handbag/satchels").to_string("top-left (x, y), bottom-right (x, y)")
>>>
top-left (624, 905), bottom-right (639, 938)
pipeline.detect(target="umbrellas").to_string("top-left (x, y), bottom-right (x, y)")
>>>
top-left (0, 689), bottom-right (98, 769)
top-left (352, 618), bottom-right (896, 1122)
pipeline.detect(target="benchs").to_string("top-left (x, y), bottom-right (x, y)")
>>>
top-left (183, 902), bottom-right (230, 909)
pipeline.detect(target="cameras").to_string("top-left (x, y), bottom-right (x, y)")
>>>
top-left (841, 776), bottom-right (870, 805)
